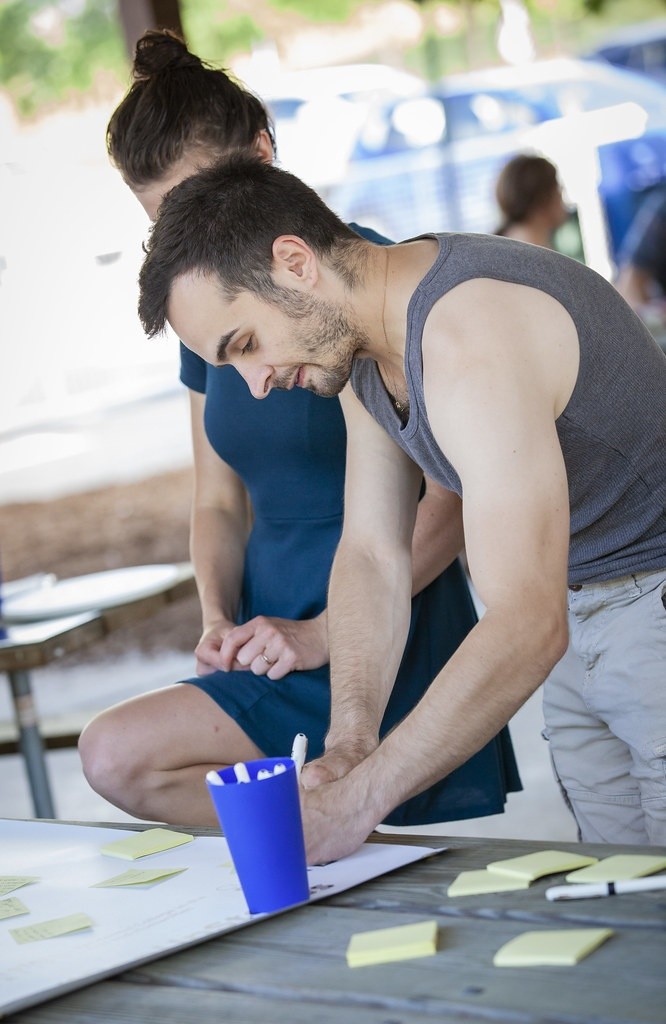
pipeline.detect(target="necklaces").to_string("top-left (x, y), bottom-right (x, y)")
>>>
top-left (381, 246), bottom-right (402, 409)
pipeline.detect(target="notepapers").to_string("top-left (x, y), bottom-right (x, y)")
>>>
top-left (447, 870), bottom-right (530, 898)
top-left (493, 927), bottom-right (615, 967)
top-left (486, 849), bottom-right (599, 883)
top-left (100, 827), bottom-right (194, 861)
top-left (564, 853), bottom-right (666, 883)
top-left (345, 919), bottom-right (438, 969)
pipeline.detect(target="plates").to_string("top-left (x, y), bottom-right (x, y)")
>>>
top-left (1, 564), bottom-right (178, 620)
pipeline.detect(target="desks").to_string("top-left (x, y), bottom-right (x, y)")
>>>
top-left (0, 820), bottom-right (666, 1024)
top-left (0, 562), bottom-right (199, 818)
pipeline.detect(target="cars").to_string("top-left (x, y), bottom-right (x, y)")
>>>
top-left (252, 30), bottom-right (666, 268)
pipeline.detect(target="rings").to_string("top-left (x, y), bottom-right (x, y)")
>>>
top-left (261, 653), bottom-right (273, 665)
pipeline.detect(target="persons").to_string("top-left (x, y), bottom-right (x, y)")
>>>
top-left (138, 149), bottom-right (666, 866)
top-left (494, 155), bottom-right (666, 339)
top-left (78, 29), bottom-right (523, 831)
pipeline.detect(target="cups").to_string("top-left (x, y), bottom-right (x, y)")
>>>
top-left (205, 757), bottom-right (310, 915)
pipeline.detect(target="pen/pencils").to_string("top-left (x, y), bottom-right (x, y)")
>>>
top-left (291, 732), bottom-right (309, 776)
top-left (205, 763), bottom-right (287, 787)
top-left (544, 875), bottom-right (666, 901)
